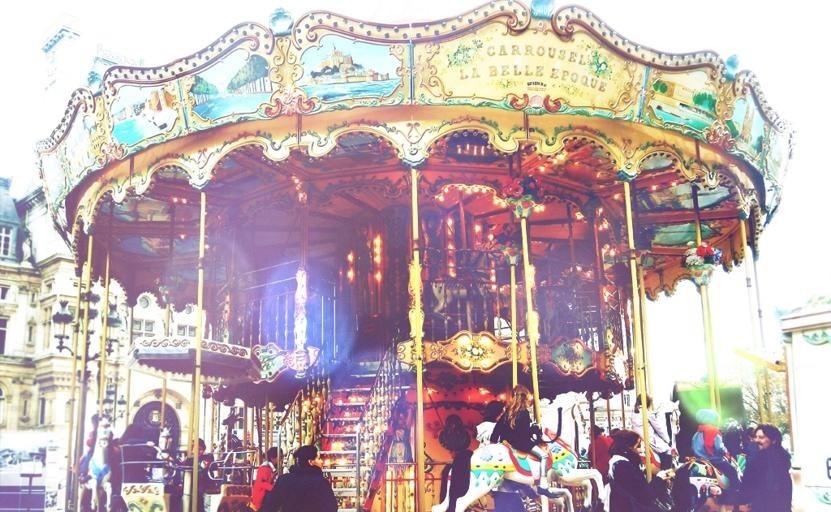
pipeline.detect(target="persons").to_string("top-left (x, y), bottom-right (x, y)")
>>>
top-left (176, 438), bottom-right (338, 511)
top-left (490, 383), bottom-right (559, 498)
top-left (588, 393), bottom-right (792, 512)
